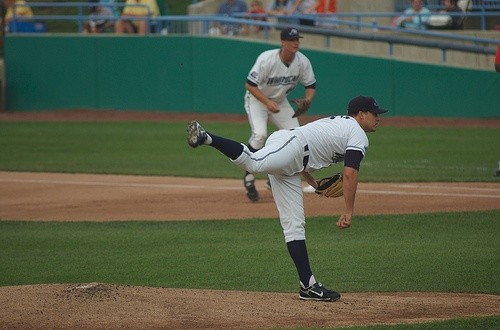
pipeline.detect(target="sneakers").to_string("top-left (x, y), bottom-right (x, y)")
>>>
top-left (299, 283), bottom-right (342, 303)
top-left (242, 173), bottom-right (261, 203)
top-left (186, 120), bottom-right (207, 149)
top-left (264, 177), bottom-right (272, 191)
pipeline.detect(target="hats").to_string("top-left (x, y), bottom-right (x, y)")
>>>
top-left (347, 96), bottom-right (388, 118)
top-left (281, 27), bottom-right (305, 41)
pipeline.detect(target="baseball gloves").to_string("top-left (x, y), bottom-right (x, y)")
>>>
top-left (291, 97), bottom-right (310, 118)
top-left (315, 173), bottom-right (344, 198)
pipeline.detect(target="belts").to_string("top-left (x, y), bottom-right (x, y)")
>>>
top-left (303, 145), bottom-right (310, 169)
